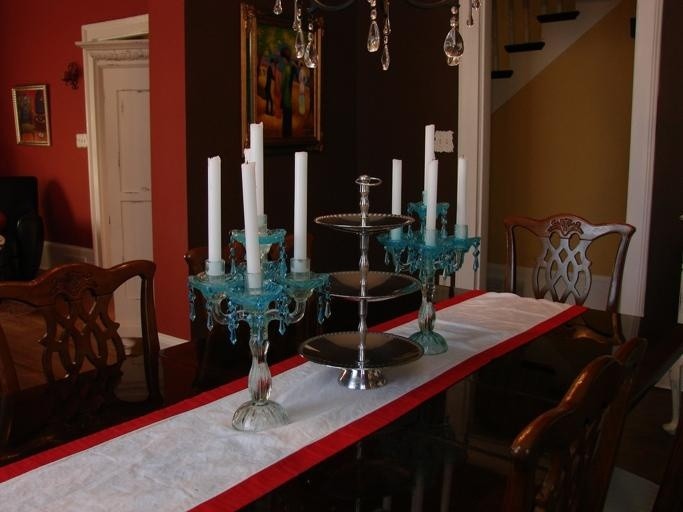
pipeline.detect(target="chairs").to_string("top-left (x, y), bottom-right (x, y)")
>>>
top-left (1, 259), bottom-right (164, 466)
top-left (429, 338), bottom-right (650, 511)
top-left (501, 214), bottom-right (636, 311)
top-left (0, 175), bottom-right (44, 282)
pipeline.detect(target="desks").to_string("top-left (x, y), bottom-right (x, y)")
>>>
top-left (1, 281), bottom-right (665, 509)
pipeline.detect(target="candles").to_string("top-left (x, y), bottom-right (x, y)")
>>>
top-left (391, 125), bottom-right (468, 247)
top-left (206, 122), bottom-right (312, 289)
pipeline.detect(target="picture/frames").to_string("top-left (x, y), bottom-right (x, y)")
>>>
top-left (239, 24), bottom-right (319, 150)
top-left (11, 84), bottom-right (50, 147)
